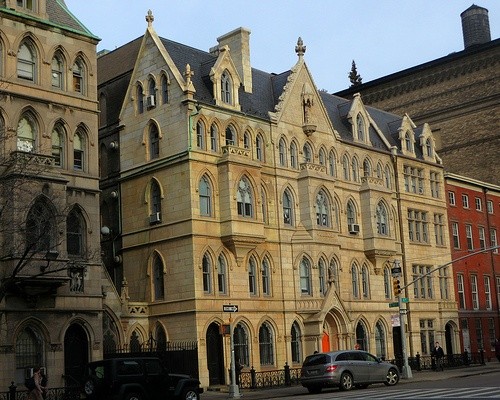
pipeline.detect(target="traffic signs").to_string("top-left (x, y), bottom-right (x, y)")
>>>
top-left (223, 305), bottom-right (238, 312)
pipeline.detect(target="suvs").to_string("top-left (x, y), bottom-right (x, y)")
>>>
top-left (83, 358), bottom-right (202, 400)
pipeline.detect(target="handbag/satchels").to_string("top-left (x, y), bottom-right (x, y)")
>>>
top-left (24, 374), bottom-right (37, 391)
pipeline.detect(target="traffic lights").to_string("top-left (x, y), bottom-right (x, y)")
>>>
top-left (393, 279), bottom-right (401, 297)
top-left (222, 324), bottom-right (231, 336)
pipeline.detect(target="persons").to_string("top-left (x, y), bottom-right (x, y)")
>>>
top-left (31, 368), bottom-right (48, 400)
top-left (430, 342), bottom-right (445, 372)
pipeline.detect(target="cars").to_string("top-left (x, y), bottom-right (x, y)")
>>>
top-left (300, 350), bottom-right (400, 394)
